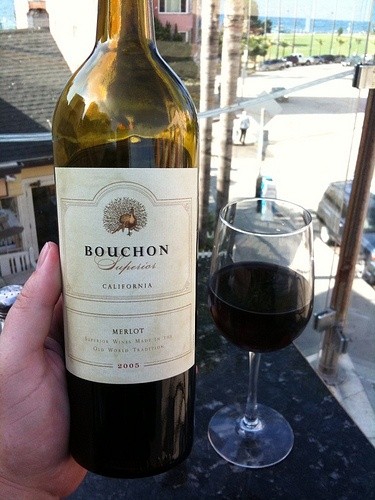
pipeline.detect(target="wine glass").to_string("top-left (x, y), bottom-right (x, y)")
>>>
top-left (208, 194), bottom-right (314, 468)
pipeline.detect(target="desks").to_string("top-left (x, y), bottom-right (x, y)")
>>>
top-left (55, 254), bottom-right (374, 499)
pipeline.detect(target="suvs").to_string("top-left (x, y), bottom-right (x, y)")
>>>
top-left (316, 181), bottom-right (375, 284)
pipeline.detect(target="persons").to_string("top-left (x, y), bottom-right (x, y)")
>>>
top-left (236, 110), bottom-right (250, 146)
top-left (0, 239), bottom-right (90, 500)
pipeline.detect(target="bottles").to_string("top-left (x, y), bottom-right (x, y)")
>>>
top-left (0, 282), bottom-right (26, 331)
top-left (52, 0), bottom-right (204, 479)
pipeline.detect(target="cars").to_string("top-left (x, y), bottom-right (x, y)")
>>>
top-left (255, 54), bottom-right (374, 71)
